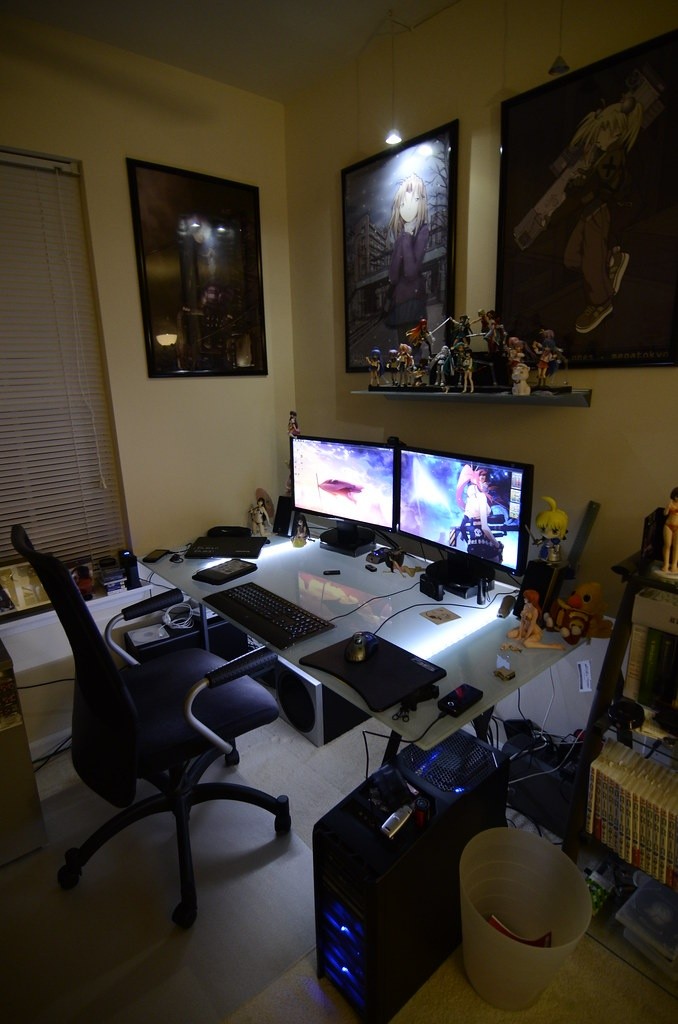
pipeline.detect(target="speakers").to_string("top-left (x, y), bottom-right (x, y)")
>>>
top-left (276, 655), bottom-right (372, 747)
top-left (512, 560), bottom-right (565, 627)
top-left (273, 495), bottom-right (300, 537)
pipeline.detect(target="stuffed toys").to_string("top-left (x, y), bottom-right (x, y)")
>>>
top-left (545, 582), bottom-right (613, 645)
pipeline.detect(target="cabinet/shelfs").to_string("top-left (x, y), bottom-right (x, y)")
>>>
top-left (0, 639), bottom-right (49, 872)
top-left (560, 548), bottom-right (678, 1000)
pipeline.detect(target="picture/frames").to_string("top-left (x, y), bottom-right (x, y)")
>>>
top-left (63, 555), bottom-right (93, 602)
top-left (339, 118), bottom-right (460, 375)
top-left (0, 551), bottom-right (56, 625)
top-left (496, 28), bottom-right (678, 370)
top-left (125, 157), bottom-right (270, 380)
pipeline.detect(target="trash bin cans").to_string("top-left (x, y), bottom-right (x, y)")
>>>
top-left (459, 828), bottom-right (594, 1012)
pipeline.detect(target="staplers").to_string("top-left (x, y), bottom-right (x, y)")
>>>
top-left (206, 526), bottom-right (253, 536)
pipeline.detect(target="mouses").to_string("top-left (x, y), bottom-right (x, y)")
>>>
top-left (345, 632), bottom-right (380, 661)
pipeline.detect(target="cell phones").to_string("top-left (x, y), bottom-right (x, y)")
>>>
top-left (143, 548), bottom-right (169, 563)
top-left (438, 684), bottom-right (484, 717)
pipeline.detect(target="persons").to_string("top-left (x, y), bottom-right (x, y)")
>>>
top-left (296, 514), bottom-right (310, 539)
top-left (660, 487), bottom-right (678, 572)
top-left (250, 498), bottom-right (272, 537)
top-left (476, 309), bottom-right (557, 385)
top-left (366, 350), bottom-right (385, 387)
top-left (533, 496), bottom-right (569, 562)
top-left (387, 344), bottom-right (428, 386)
top-left (429, 315), bottom-right (476, 392)
top-left (506, 590), bottom-right (565, 649)
top-left (404, 319), bottom-right (432, 354)
top-left (287, 411), bottom-right (298, 439)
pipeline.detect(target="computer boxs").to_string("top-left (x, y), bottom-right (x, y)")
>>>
top-left (316, 728), bottom-right (511, 1024)
top-left (124, 611), bottom-right (250, 662)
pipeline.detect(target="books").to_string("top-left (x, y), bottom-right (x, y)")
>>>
top-left (585, 738), bottom-right (678, 892)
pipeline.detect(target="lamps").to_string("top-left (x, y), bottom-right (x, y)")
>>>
top-left (548, 0), bottom-right (570, 77)
top-left (384, 10), bottom-right (403, 145)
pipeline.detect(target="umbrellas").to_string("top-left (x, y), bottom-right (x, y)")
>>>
top-left (248, 488), bottom-right (275, 518)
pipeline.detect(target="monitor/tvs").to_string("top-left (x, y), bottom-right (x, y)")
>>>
top-left (288, 434), bottom-right (534, 600)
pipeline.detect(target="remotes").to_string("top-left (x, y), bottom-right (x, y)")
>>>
top-left (497, 595), bottom-right (517, 619)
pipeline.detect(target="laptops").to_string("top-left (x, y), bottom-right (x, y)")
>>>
top-left (185, 536), bottom-right (268, 560)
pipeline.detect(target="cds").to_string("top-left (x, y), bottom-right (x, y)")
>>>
top-left (132, 626), bottom-right (165, 642)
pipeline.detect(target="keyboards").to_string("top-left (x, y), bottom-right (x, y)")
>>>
top-left (202, 582), bottom-right (336, 652)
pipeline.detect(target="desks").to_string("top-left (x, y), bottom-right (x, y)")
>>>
top-left (136, 520), bottom-right (590, 772)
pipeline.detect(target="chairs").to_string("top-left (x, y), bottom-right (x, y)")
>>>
top-left (9, 523), bottom-right (290, 930)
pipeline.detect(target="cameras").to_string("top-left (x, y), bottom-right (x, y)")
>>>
top-left (419, 574), bottom-right (445, 602)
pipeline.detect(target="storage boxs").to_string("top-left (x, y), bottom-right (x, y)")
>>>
top-left (615, 878), bottom-right (678, 961)
top-left (621, 928), bottom-right (678, 982)
top-left (630, 586), bottom-right (678, 636)
top-left (118, 549), bottom-right (142, 591)
